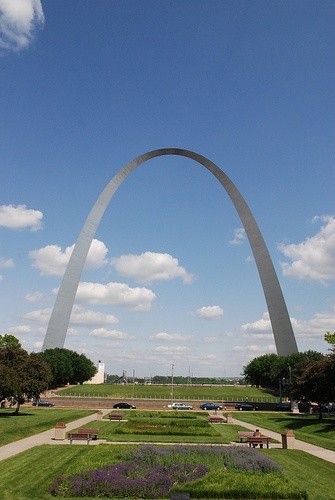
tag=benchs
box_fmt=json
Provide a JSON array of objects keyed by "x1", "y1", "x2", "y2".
[
  {"x1": 238, "y1": 431, "x2": 254, "y2": 443},
  {"x1": 79, "y1": 428, "x2": 100, "y2": 440},
  {"x1": 208, "y1": 415, "x2": 227, "y2": 424},
  {"x1": 247, "y1": 435, "x2": 272, "y2": 449},
  {"x1": 68, "y1": 433, "x2": 92, "y2": 445},
  {"x1": 109, "y1": 414, "x2": 122, "y2": 421}
]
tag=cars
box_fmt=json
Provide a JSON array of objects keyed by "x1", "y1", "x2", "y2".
[
  {"x1": 235, "y1": 401, "x2": 259, "y2": 411},
  {"x1": 200, "y1": 402, "x2": 224, "y2": 411},
  {"x1": 272, "y1": 403, "x2": 291, "y2": 411},
  {"x1": 113, "y1": 402, "x2": 137, "y2": 409},
  {"x1": 32, "y1": 401, "x2": 56, "y2": 408},
  {"x1": 171, "y1": 402, "x2": 193, "y2": 410},
  {"x1": 299, "y1": 402, "x2": 335, "y2": 413}
]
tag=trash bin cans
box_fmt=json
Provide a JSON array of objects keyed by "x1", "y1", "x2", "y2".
[
  {"x1": 55, "y1": 422, "x2": 66, "y2": 439},
  {"x1": 97, "y1": 410, "x2": 103, "y2": 420},
  {"x1": 227, "y1": 413, "x2": 232, "y2": 423},
  {"x1": 281, "y1": 430, "x2": 295, "y2": 449}
]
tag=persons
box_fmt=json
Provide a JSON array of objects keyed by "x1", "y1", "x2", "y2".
[{"x1": 252, "y1": 429, "x2": 263, "y2": 448}]
